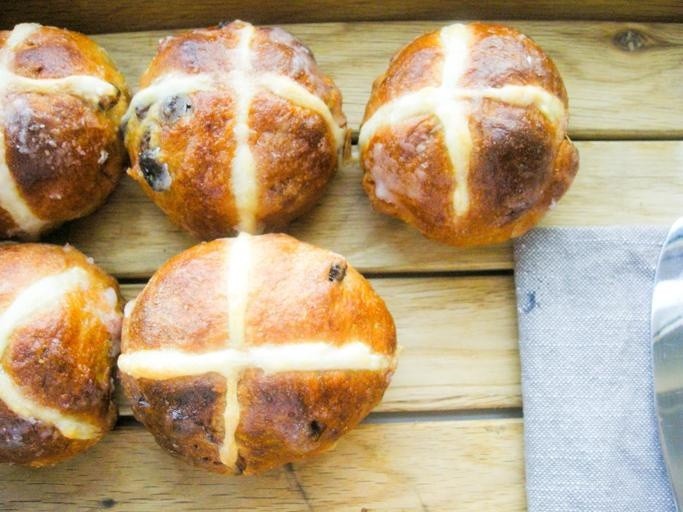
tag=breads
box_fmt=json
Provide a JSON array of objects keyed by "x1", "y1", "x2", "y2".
[
  {"x1": 358, "y1": 21, "x2": 581, "y2": 247},
  {"x1": 116, "y1": 233, "x2": 397, "y2": 478},
  {"x1": 0, "y1": 22, "x2": 132, "y2": 240},
  {"x1": 0, "y1": 241, "x2": 126, "y2": 469},
  {"x1": 122, "y1": 19, "x2": 353, "y2": 242}
]
